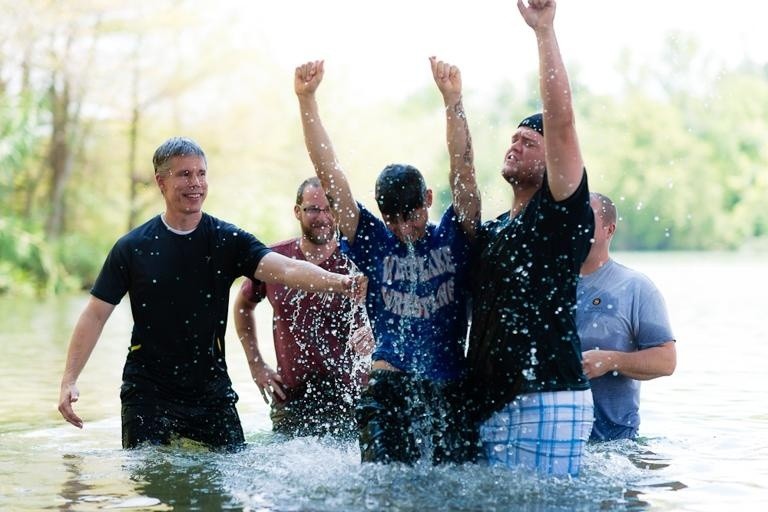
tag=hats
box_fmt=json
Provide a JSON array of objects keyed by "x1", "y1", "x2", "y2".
[{"x1": 518, "y1": 113, "x2": 543, "y2": 135}]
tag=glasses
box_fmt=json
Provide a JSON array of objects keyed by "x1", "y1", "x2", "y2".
[{"x1": 301, "y1": 204, "x2": 331, "y2": 214}]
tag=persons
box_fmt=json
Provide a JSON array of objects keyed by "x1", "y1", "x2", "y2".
[
  {"x1": 233, "y1": 174, "x2": 376, "y2": 441},
  {"x1": 293, "y1": 54, "x2": 482, "y2": 465},
  {"x1": 461, "y1": 0, "x2": 598, "y2": 482},
  {"x1": 570, "y1": 192, "x2": 681, "y2": 442},
  {"x1": 56, "y1": 135, "x2": 370, "y2": 453}
]
